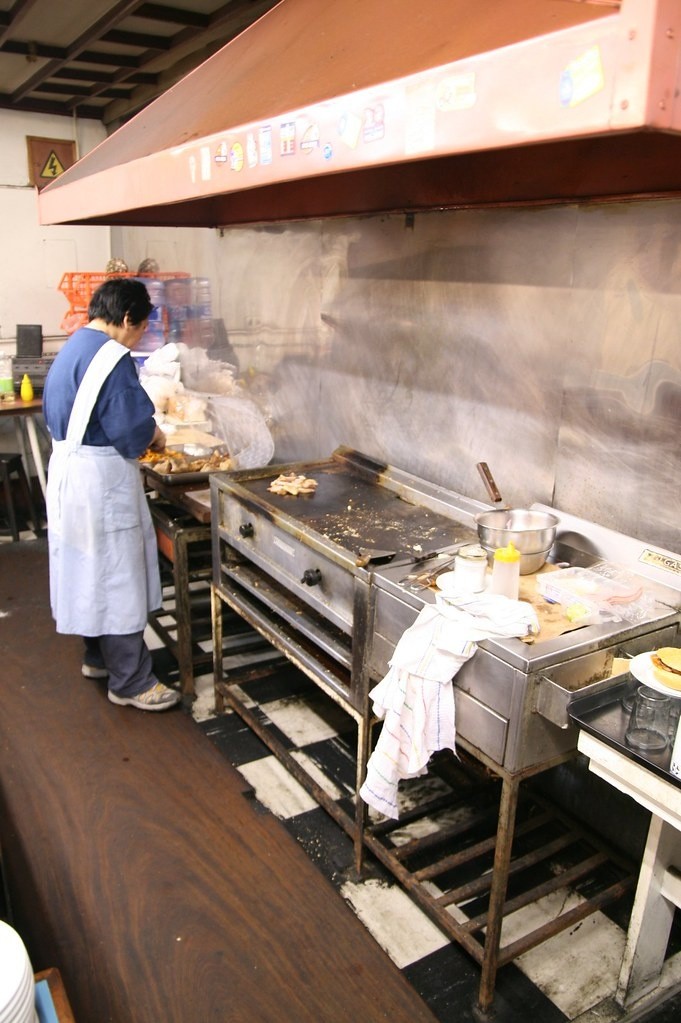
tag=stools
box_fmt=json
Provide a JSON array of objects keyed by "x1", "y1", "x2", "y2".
[{"x1": 0, "y1": 453, "x2": 40, "y2": 542}]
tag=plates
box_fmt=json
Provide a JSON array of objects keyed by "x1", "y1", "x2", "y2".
[
  {"x1": 436, "y1": 572, "x2": 492, "y2": 603},
  {"x1": 627, "y1": 650, "x2": 681, "y2": 701}
]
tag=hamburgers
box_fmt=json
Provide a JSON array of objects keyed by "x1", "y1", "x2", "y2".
[{"x1": 650, "y1": 647, "x2": 681, "y2": 693}]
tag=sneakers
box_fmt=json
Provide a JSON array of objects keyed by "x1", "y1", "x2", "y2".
[
  {"x1": 107, "y1": 682, "x2": 181, "y2": 711},
  {"x1": 81, "y1": 664, "x2": 109, "y2": 678}
]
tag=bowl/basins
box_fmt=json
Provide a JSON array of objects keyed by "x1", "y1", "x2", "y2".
[
  {"x1": 474, "y1": 508, "x2": 561, "y2": 554},
  {"x1": 480, "y1": 543, "x2": 556, "y2": 574}
]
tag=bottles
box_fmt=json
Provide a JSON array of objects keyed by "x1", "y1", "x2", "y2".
[
  {"x1": 454, "y1": 544, "x2": 487, "y2": 593},
  {"x1": 490, "y1": 541, "x2": 520, "y2": 603},
  {"x1": 162, "y1": 277, "x2": 211, "y2": 346},
  {"x1": 21, "y1": 373, "x2": 34, "y2": 402},
  {"x1": 119, "y1": 277, "x2": 164, "y2": 375}
]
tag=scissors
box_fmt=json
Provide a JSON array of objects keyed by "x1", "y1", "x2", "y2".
[{"x1": 397, "y1": 556, "x2": 455, "y2": 592}]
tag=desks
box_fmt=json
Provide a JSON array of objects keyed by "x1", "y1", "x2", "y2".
[
  {"x1": 576, "y1": 730, "x2": 681, "y2": 1014},
  {"x1": 0, "y1": 390, "x2": 47, "y2": 510}
]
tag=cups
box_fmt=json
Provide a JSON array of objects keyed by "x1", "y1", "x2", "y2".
[{"x1": 623, "y1": 675, "x2": 681, "y2": 750}]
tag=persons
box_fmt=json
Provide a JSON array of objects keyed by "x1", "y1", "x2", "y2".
[{"x1": 43, "y1": 278, "x2": 181, "y2": 710}]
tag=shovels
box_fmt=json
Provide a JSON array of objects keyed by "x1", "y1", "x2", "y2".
[
  {"x1": 410, "y1": 542, "x2": 471, "y2": 564},
  {"x1": 355, "y1": 547, "x2": 396, "y2": 567}
]
